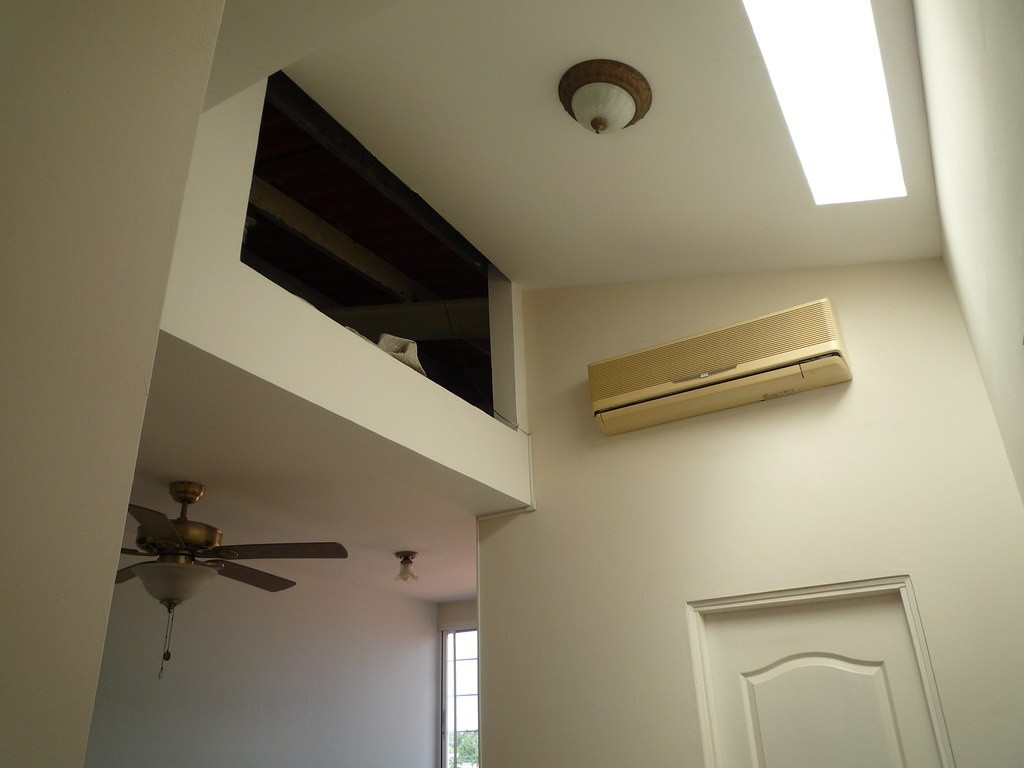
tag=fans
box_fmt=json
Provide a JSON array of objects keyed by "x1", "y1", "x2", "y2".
[{"x1": 115, "y1": 479, "x2": 350, "y2": 594}]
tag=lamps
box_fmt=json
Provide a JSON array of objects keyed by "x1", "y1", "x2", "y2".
[
  {"x1": 558, "y1": 58, "x2": 653, "y2": 136},
  {"x1": 392, "y1": 551, "x2": 420, "y2": 583},
  {"x1": 131, "y1": 561, "x2": 217, "y2": 682}
]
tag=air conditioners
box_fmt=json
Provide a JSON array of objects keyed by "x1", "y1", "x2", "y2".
[{"x1": 586, "y1": 298, "x2": 853, "y2": 436}]
